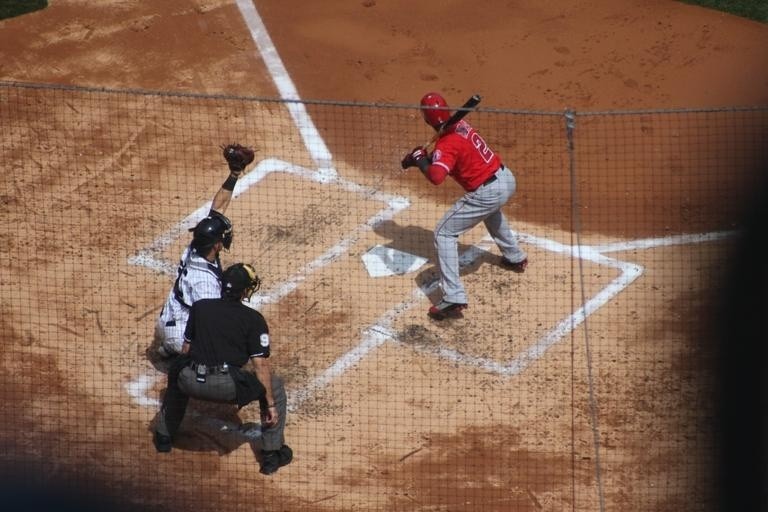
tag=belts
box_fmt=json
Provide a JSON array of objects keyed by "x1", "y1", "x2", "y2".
[
  {"x1": 188, "y1": 360, "x2": 222, "y2": 376},
  {"x1": 483, "y1": 164, "x2": 504, "y2": 185}
]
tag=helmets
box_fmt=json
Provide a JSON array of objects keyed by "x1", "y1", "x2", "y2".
[
  {"x1": 420, "y1": 93, "x2": 451, "y2": 127},
  {"x1": 221, "y1": 263, "x2": 261, "y2": 303},
  {"x1": 194, "y1": 209, "x2": 234, "y2": 250}
]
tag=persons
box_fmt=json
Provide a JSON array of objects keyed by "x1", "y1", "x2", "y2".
[
  {"x1": 401, "y1": 92, "x2": 529, "y2": 318},
  {"x1": 157, "y1": 143, "x2": 255, "y2": 357},
  {"x1": 152, "y1": 263, "x2": 293, "y2": 476}
]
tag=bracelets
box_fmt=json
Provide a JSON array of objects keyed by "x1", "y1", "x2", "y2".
[{"x1": 418, "y1": 158, "x2": 430, "y2": 172}]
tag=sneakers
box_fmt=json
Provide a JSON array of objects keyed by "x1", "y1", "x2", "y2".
[
  {"x1": 501, "y1": 255, "x2": 527, "y2": 270},
  {"x1": 154, "y1": 432, "x2": 171, "y2": 452},
  {"x1": 429, "y1": 300, "x2": 468, "y2": 318},
  {"x1": 262, "y1": 445, "x2": 292, "y2": 473}
]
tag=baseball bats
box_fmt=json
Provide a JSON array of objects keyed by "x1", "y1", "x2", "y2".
[{"x1": 422, "y1": 94, "x2": 481, "y2": 150}]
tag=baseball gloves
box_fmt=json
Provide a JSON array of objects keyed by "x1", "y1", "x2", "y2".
[{"x1": 219, "y1": 142, "x2": 260, "y2": 178}]
